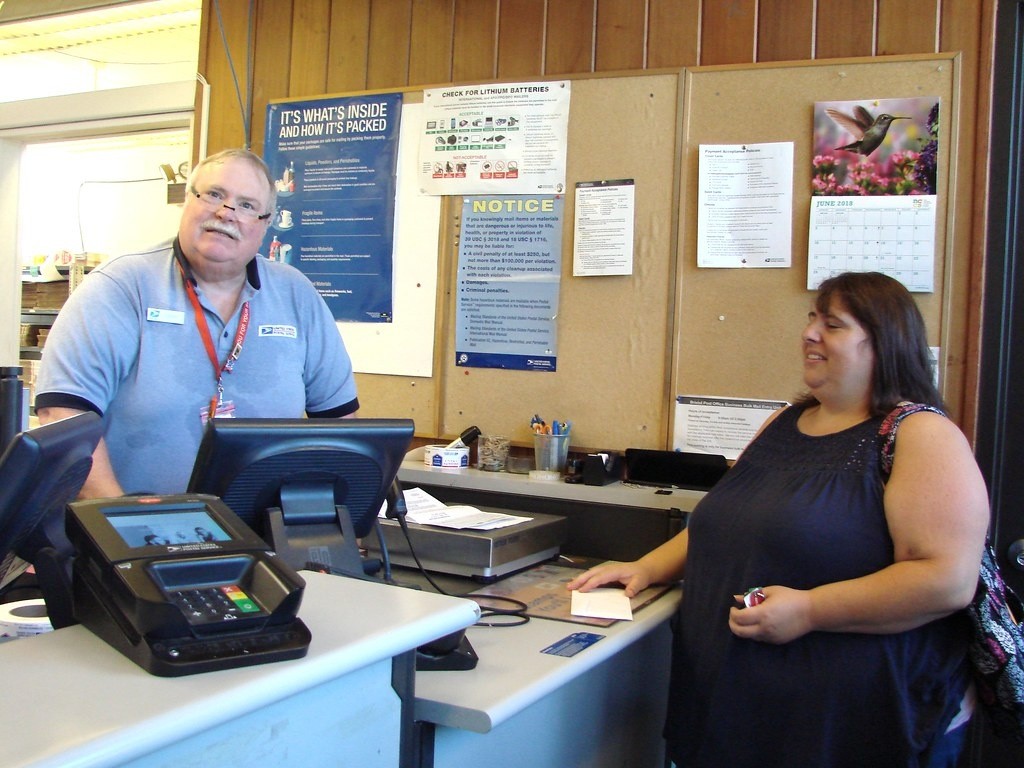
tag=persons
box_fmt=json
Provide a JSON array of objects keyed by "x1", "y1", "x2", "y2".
[
  {"x1": 565, "y1": 269, "x2": 1024, "y2": 768},
  {"x1": 34, "y1": 149, "x2": 361, "y2": 508}
]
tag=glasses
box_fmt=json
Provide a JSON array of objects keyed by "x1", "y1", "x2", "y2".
[{"x1": 190, "y1": 181, "x2": 273, "y2": 222}]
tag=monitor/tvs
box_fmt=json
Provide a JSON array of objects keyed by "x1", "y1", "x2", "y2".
[
  {"x1": 0, "y1": 410, "x2": 105, "y2": 633},
  {"x1": 186, "y1": 418, "x2": 415, "y2": 582}
]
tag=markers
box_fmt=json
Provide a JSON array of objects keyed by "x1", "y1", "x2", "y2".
[{"x1": 445, "y1": 426, "x2": 481, "y2": 448}]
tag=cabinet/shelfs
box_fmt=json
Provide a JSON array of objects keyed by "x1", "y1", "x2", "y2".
[{"x1": 18, "y1": 266, "x2": 95, "y2": 360}]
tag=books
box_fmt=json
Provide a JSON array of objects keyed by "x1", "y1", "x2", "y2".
[
  {"x1": 16, "y1": 356, "x2": 41, "y2": 406},
  {"x1": 27, "y1": 414, "x2": 41, "y2": 431},
  {"x1": 21, "y1": 273, "x2": 70, "y2": 311},
  {"x1": 18, "y1": 323, "x2": 52, "y2": 349}
]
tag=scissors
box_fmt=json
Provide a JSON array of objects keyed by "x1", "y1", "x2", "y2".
[{"x1": 534, "y1": 423, "x2": 552, "y2": 435}]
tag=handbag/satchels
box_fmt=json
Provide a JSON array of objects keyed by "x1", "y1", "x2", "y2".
[{"x1": 877, "y1": 401, "x2": 1024, "y2": 744}]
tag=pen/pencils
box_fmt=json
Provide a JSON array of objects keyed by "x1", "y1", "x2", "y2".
[
  {"x1": 530, "y1": 413, "x2": 573, "y2": 435},
  {"x1": 210, "y1": 394, "x2": 218, "y2": 418}
]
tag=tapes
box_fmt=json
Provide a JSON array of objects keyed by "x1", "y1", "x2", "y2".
[
  {"x1": 0, "y1": 599, "x2": 55, "y2": 638},
  {"x1": 423, "y1": 444, "x2": 471, "y2": 468}
]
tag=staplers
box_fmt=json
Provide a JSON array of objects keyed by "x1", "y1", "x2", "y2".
[{"x1": 564, "y1": 459, "x2": 585, "y2": 484}]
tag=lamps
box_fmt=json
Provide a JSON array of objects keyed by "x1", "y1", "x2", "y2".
[{"x1": 159, "y1": 163, "x2": 177, "y2": 183}]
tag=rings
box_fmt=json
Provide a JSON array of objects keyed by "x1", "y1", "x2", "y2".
[{"x1": 744, "y1": 585, "x2": 764, "y2": 595}]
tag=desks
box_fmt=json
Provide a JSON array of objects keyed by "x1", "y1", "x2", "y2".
[
  {"x1": 396, "y1": 461, "x2": 710, "y2": 562},
  {"x1": 377, "y1": 565, "x2": 682, "y2": 768},
  {"x1": 0, "y1": 571, "x2": 480, "y2": 768}
]
